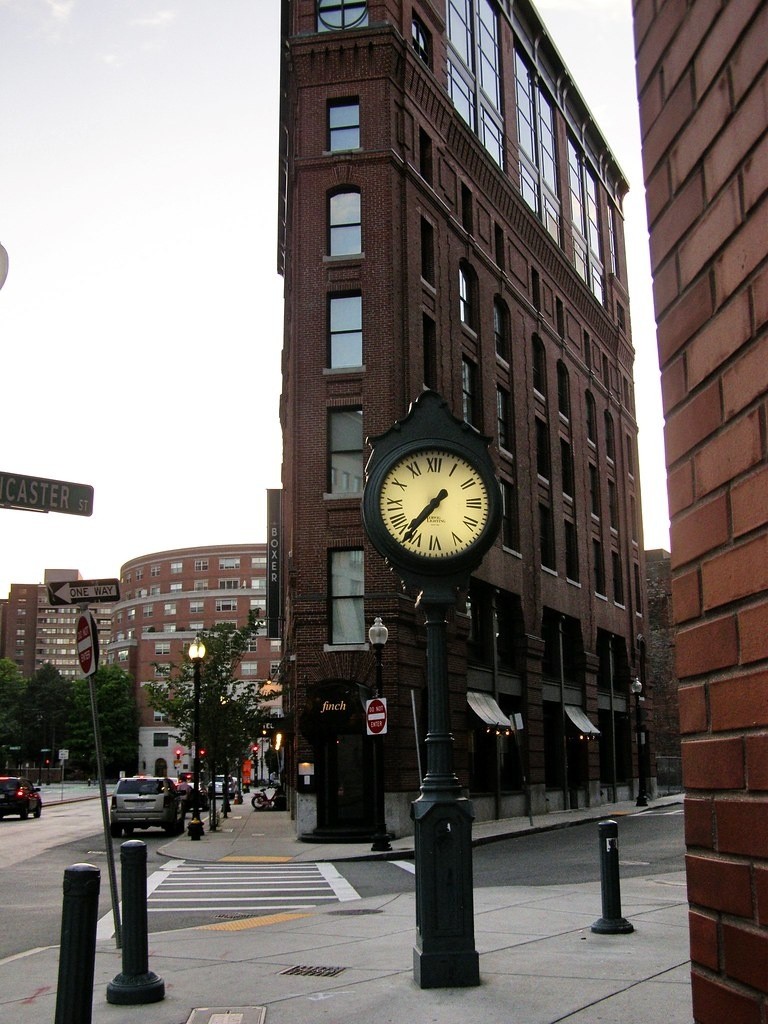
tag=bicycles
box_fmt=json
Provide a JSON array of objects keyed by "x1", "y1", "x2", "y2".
[{"x1": 251, "y1": 785, "x2": 285, "y2": 809}]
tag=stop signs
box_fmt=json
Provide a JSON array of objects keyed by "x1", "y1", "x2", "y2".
[
  {"x1": 365, "y1": 698, "x2": 389, "y2": 736},
  {"x1": 75, "y1": 612, "x2": 97, "y2": 680}
]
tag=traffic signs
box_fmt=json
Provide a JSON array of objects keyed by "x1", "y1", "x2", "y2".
[
  {"x1": 0, "y1": 471, "x2": 94, "y2": 517},
  {"x1": 47, "y1": 578, "x2": 122, "y2": 608}
]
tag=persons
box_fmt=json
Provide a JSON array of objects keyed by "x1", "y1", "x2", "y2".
[{"x1": 176, "y1": 776, "x2": 191, "y2": 818}]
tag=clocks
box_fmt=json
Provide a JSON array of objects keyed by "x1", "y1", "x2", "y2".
[{"x1": 360, "y1": 437, "x2": 503, "y2": 573}]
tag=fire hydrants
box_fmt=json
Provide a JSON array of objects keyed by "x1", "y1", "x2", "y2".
[
  {"x1": 189, "y1": 818, "x2": 201, "y2": 840},
  {"x1": 88, "y1": 778, "x2": 91, "y2": 786}
]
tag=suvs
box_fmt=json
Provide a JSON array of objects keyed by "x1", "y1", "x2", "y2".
[
  {"x1": 208, "y1": 775, "x2": 235, "y2": 798},
  {"x1": 0, "y1": 776, "x2": 42, "y2": 820},
  {"x1": 110, "y1": 777, "x2": 185, "y2": 837}
]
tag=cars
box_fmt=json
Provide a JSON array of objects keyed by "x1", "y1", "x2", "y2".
[{"x1": 185, "y1": 782, "x2": 208, "y2": 810}]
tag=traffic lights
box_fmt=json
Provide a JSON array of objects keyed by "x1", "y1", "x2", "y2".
[
  {"x1": 177, "y1": 750, "x2": 180, "y2": 759},
  {"x1": 253, "y1": 746, "x2": 259, "y2": 758},
  {"x1": 46, "y1": 759, "x2": 50, "y2": 767},
  {"x1": 200, "y1": 750, "x2": 205, "y2": 760}
]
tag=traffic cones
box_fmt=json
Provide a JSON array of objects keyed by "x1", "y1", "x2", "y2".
[{"x1": 233, "y1": 791, "x2": 239, "y2": 805}]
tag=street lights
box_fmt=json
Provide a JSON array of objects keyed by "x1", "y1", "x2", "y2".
[
  {"x1": 629, "y1": 677, "x2": 649, "y2": 807},
  {"x1": 368, "y1": 612, "x2": 393, "y2": 852},
  {"x1": 187, "y1": 632, "x2": 205, "y2": 833}
]
